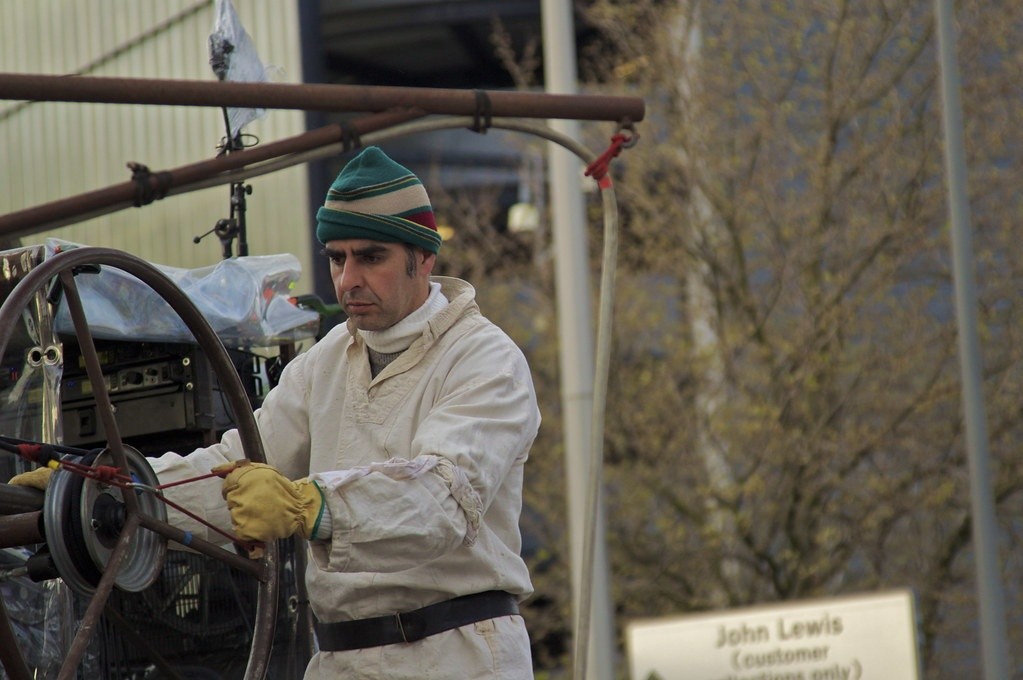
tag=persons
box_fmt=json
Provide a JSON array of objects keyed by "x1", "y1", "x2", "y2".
[{"x1": 11, "y1": 146, "x2": 540, "y2": 679}]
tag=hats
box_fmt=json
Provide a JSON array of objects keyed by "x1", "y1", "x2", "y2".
[{"x1": 314, "y1": 146, "x2": 443, "y2": 258}]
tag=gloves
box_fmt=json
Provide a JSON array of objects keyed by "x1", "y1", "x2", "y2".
[
  {"x1": 5, "y1": 465, "x2": 54, "y2": 491},
  {"x1": 210, "y1": 459, "x2": 325, "y2": 551}
]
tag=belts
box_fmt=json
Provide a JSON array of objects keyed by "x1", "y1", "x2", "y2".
[{"x1": 311, "y1": 589, "x2": 520, "y2": 653}]
